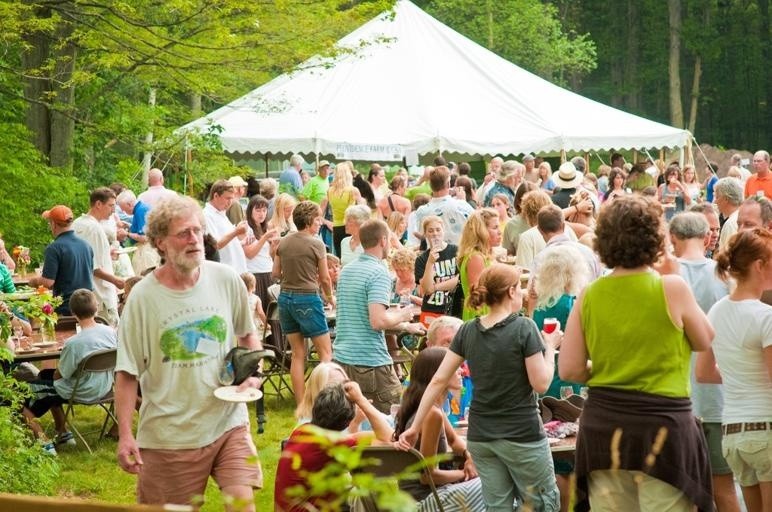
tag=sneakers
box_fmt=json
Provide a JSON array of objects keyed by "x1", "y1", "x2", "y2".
[
  {"x1": 103, "y1": 432, "x2": 119, "y2": 441},
  {"x1": 45, "y1": 444, "x2": 57, "y2": 456},
  {"x1": 55, "y1": 431, "x2": 76, "y2": 447}
]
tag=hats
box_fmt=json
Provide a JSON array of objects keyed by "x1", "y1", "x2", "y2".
[
  {"x1": 319, "y1": 160, "x2": 329, "y2": 168},
  {"x1": 217, "y1": 348, "x2": 276, "y2": 386},
  {"x1": 552, "y1": 161, "x2": 583, "y2": 189},
  {"x1": 228, "y1": 176, "x2": 248, "y2": 187},
  {"x1": 43, "y1": 205, "x2": 73, "y2": 224},
  {"x1": 538, "y1": 394, "x2": 585, "y2": 423}
]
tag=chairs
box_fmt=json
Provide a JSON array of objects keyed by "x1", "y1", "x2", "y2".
[
  {"x1": 6, "y1": 267, "x2": 118, "y2": 455},
  {"x1": 259, "y1": 301, "x2": 296, "y2": 408},
  {"x1": 350, "y1": 448, "x2": 446, "y2": 512}
]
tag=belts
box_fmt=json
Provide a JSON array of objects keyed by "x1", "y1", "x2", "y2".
[{"x1": 722, "y1": 422, "x2": 772, "y2": 434}]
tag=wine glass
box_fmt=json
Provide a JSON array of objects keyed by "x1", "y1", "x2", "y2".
[
  {"x1": 464, "y1": 406, "x2": 469, "y2": 422},
  {"x1": 580, "y1": 386, "x2": 590, "y2": 400},
  {"x1": 559, "y1": 385, "x2": 574, "y2": 400},
  {"x1": 216, "y1": 361, "x2": 235, "y2": 386},
  {"x1": 543, "y1": 318, "x2": 560, "y2": 354},
  {"x1": 13, "y1": 326, "x2": 23, "y2": 344}
]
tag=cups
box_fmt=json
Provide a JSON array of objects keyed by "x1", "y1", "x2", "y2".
[
  {"x1": 431, "y1": 238, "x2": 442, "y2": 251},
  {"x1": 20, "y1": 337, "x2": 33, "y2": 350},
  {"x1": 39, "y1": 262, "x2": 45, "y2": 268},
  {"x1": 34, "y1": 267, "x2": 42, "y2": 276},
  {"x1": 399, "y1": 296, "x2": 410, "y2": 309},
  {"x1": 9, "y1": 269, "x2": 15, "y2": 276}
]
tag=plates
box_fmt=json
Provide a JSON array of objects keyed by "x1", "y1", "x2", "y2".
[
  {"x1": 213, "y1": 385, "x2": 263, "y2": 402},
  {"x1": 33, "y1": 341, "x2": 57, "y2": 347},
  {"x1": 453, "y1": 420, "x2": 469, "y2": 427},
  {"x1": 115, "y1": 247, "x2": 137, "y2": 254},
  {"x1": 16, "y1": 347, "x2": 39, "y2": 352}
]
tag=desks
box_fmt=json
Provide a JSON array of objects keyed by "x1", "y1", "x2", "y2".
[{"x1": 307, "y1": 309, "x2": 336, "y2": 364}]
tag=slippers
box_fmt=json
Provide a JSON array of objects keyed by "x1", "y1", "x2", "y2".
[{"x1": 398, "y1": 375, "x2": 406, "y2": 382}]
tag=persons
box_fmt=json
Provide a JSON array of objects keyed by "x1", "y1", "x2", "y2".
[{"x1": 1, "y1": 150, "x2": 772, "y2": 512}]
